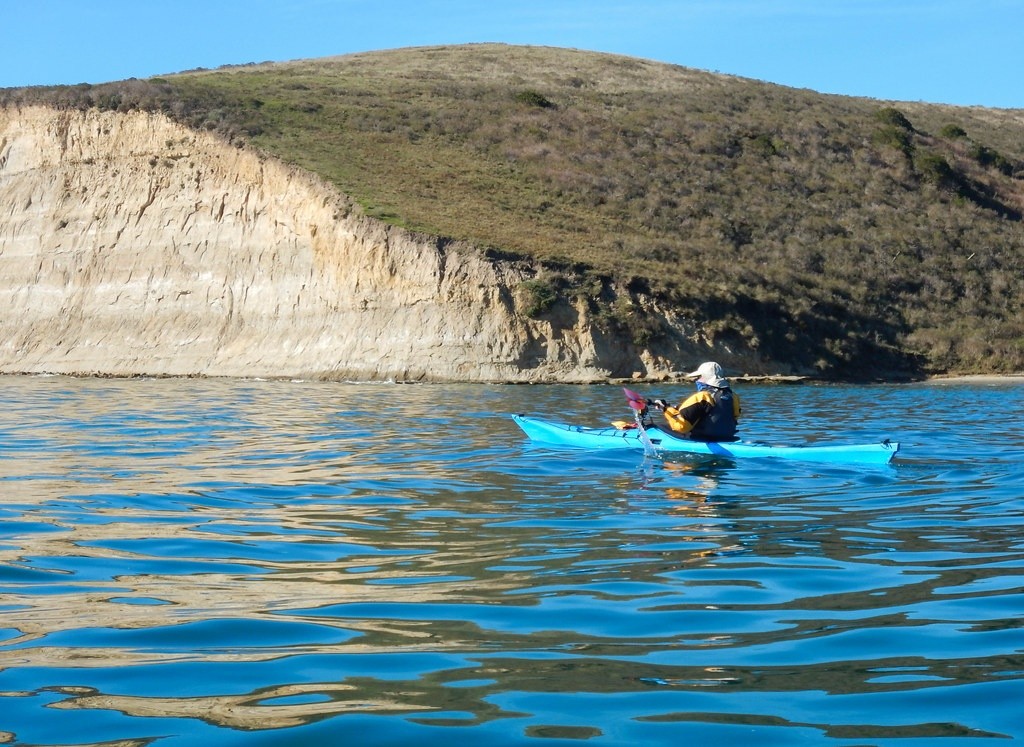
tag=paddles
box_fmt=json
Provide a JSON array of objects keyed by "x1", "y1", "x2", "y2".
[{"x1": 623, "y1": 386, "x2": 660, "y2": 410}]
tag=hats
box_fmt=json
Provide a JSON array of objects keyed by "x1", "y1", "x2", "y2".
[{"x1": 686, "y1": 362, "x2": 730, "y2": 388}]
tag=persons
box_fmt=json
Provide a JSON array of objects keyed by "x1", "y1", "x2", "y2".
[{"x1": 655, "y1": 362, "x2": 741, "y2": 441}]
